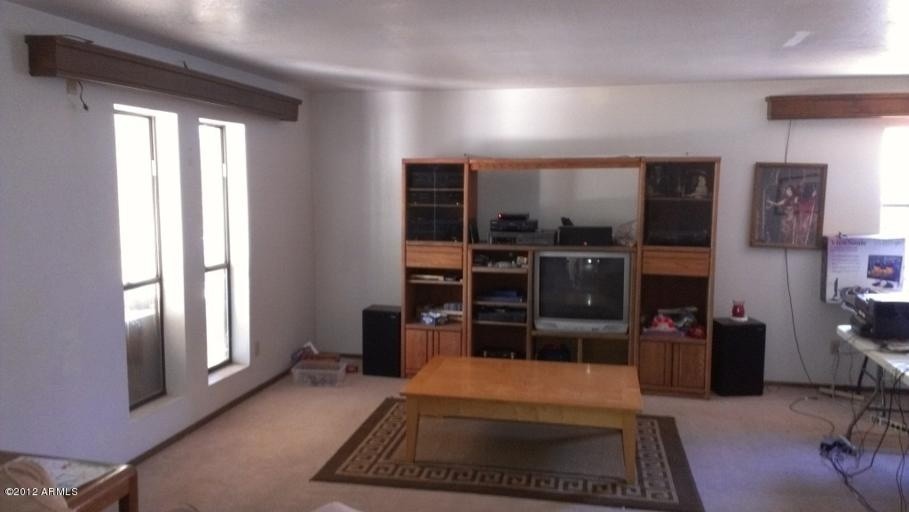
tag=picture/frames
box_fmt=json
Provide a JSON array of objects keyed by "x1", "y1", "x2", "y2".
[{"x1": 747, "y1": 161, "x2": 828, "y2": 250}]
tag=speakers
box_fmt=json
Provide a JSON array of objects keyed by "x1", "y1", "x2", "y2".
[
  {"x1": 361, "y1": 303, "x2": 402, "y2": 376},
  {"x1": 711, "y1": 315, "x2": 766, "y2": 397}
]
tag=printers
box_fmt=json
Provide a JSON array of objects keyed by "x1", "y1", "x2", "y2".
[{"x1": 850, "y1": 291, "x2": 909, "y2": 339}]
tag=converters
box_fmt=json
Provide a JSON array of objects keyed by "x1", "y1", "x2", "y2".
[{"x1": 820, "y1": 436, "x2": 835, "y2": 449}]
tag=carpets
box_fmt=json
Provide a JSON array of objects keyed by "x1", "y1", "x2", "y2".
[{"x1": 311, "y1": 396, "x2": 706, "y2": 512}]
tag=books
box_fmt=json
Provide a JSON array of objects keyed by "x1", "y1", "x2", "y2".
[{"x1": 473, "y1": 283, "x2": 526, "y2": 305}]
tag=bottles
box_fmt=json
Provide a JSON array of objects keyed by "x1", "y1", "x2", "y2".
[{"x1": 732, "y1": 301, "x2": 745, "y2": 317}]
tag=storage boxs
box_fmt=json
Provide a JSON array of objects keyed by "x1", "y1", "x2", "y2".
[{"x1": 290, "y1": 359, "x2": 348, "y2": 389}]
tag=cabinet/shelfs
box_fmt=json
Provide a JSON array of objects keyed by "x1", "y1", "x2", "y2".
[{"x1": 396, "y1": 155, "x2": 724, "y2": 402}]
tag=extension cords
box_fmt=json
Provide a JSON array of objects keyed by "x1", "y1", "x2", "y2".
[{"x1": 836, "y1": 436, "x2": 857, "y2": 457}]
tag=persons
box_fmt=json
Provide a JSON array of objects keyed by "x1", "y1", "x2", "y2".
[{"x1": 765, "y1": 182, "x2": 799, "y2": 245}]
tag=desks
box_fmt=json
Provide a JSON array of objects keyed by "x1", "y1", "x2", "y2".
[
  {"x1": 398, "y1": 355, "x2": 644, "y2": 486},
  {"x1": 1, "y1": 450, "x2": 139, "y2": 512},
  {"x1": 834, "y1": 323, "x2": 909, "y2": 456}
]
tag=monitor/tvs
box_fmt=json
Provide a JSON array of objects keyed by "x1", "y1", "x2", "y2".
[{"x1": 532, "y1": 249, "x2": 630, "y2": 334}]
